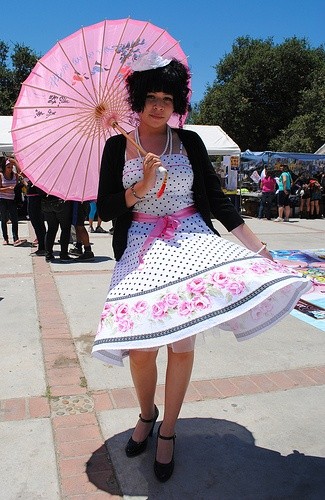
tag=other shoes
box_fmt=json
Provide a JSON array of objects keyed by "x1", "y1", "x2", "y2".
[
  {"x1": 3, "y1": 241, "x2": 9, "y2": 245},
  {"x1": 306, "y1": 212, "x2": 310, "y2": 217},
  {"x1": 71, "y1": 248, "x2": 82, "y2": 256},
  {"x1": 73, "y1": 251, "x2": 95, "y2": 261},
  {"x1": 46, "y1": 255, "x2": 55, "y2": 262},
  {"x1": 58, "y1": 239, "x2": 73, "y2": 244},
  {"x1": 96, "y1": 226, "x2": 106, "y2": 233},
  {"x1": 299, "y1": 213, "x2": 303, "y2": 219},
  {"x1": 32, "y1": 239, "x2": 39, "y2": 247},
  {"x1": 31, "y1": 248, "x2": 46, "y2": 256},
  {"x1": 273, "y1": 217, "x2": 285, "y2": 222},
  {"x1": 88, "y1": 227, "x2": 94, "y2": 233},
  {"x1": 14, "y1": 239, "x2": 27, "y2": 247},
  {"x1": 282, "y1": 217, "x2": 289, "y2": 222},
  {"x1": 60, "y1": 251, "x2": 74, "y2": 261}
]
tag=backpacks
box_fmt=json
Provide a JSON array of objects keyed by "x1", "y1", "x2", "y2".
[{"x1": 312, "y1": 183, "x2": 320, "y2": 191}]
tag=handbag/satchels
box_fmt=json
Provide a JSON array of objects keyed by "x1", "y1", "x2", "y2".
[{"x1": 250, "y1": 170, "x2": 261, "y2": 183}]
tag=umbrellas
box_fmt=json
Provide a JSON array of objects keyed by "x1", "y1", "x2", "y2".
[{"x1": 8, "y1": 15, "x2": 195, "y2": 204}]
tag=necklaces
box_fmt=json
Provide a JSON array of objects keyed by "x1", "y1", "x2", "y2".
[{"x1": 135, "y1": 125, "x2": 173, "y2": 158}]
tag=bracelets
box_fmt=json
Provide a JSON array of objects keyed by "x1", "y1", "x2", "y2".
[
  {"x1": 257, "y1": 242, "x2": 267, "y2": 254},
  {"x1": 131, "y1": 183, "x2": 141, "y2": 199}
]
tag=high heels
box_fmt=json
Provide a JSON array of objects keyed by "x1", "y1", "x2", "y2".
[
  {"x1": 125, "y1": 404, "x2": 159, "y2": 458},
  {"x1": 154, "y1": 421, "x2": 177, "y2": 483}
]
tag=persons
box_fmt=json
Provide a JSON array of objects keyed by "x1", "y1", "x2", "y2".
[
  {"x1": 258, "y1": 163, "x2": 325, "y2": 222},
  {"x1": 91, "y1": 50, "x2": 312, "y2": 483},
  {"x1": 0, "y1": 157, "x2": 114, "y2": 264}
]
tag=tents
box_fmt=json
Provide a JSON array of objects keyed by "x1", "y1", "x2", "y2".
[
  {"x1": 0, "y1": 115, "x2": 14, "y2": 154},
  {"x1": 239, "y1": 149, "x2": 325, "y2": 215},
  {"x1": 182, "y1": 125, "x2": 241, "y2": 215}
]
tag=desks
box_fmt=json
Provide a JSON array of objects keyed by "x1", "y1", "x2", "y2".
[{"x1": 242, "y1": 193, "x2": 296, "y2": 217}]
